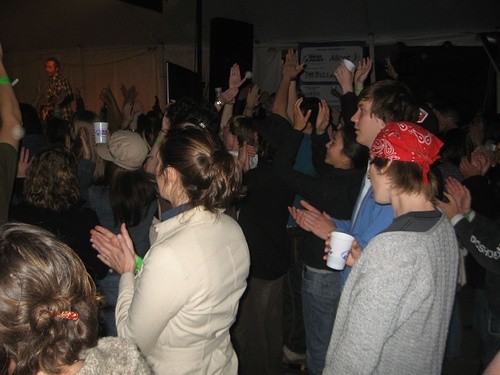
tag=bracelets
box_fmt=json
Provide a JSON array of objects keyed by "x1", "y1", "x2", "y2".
[
  {"x1": 162, "y1": 128, "x2": 168, "y2": 133},
  {"x1": 134, "y1": 256, "x2": 142, "y2": 274},
  {"x1": 355, "y1": 88, "x2": 363, "y2": 93},
  {"x1": 465, "y1": 211, "x2": 472, "y2": 220},
  {"x1": 156, "y1": 138, "x2": 164, "y2": 145},
  {"x1": 130, "y1": 128, "x2": 137, "y2": 132}
]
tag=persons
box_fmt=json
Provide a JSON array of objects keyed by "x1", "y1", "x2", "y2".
[
  {"x1": 0, "y1": 47, "x2": 500, "y2": 375},
  {"x1": 321, "y1": 122, "x2": 460, "y2": 375}
]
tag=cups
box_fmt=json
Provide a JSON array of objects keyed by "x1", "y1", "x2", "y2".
[
  {"x1": 344, "y1": 59, "x2": 355, "y2": 71},
  {"x1": 327, "y1": 231, "x2": 355, "y2": 270},
  {"x1": 93, "y1": 122, "x2": 108, "y2": 143}
]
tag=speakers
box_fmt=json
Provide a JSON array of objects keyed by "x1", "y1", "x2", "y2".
[
  {"x1": 166, "y1": 61, "x2": 203, "y2": 106},
  {"x1": 210, "y1": 18, "x2": 254, "y2": 88}
]
